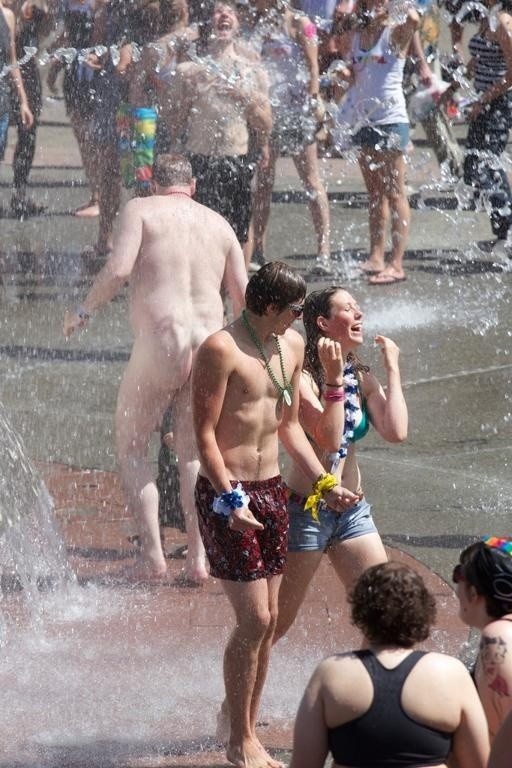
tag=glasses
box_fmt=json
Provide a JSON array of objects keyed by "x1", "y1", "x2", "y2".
[
  {"x1": 453, "y1": 565, "x2": 467, "y2": 584},
  {"x1": 284, "y1": 304, "x2": 305, "y2": 318}
]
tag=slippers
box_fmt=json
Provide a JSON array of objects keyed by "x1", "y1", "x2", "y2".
[{"x1": 312, "y1": 256, "x2": 406, "y2": 284}]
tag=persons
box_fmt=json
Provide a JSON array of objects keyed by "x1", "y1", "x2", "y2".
[{"x1": 1, "y1": 0, "x2": 512, "y2": 767}]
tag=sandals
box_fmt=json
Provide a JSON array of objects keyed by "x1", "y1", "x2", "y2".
[
  {"x1": 126, "y1": 531, "x2": 165, "y2": 552},
  {"x1": 167, "y1": 544, "x2": 188, "y2": 558}
]
